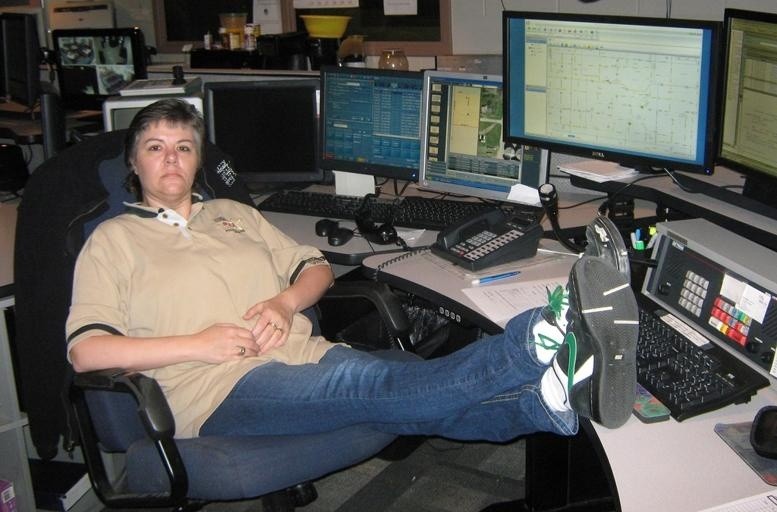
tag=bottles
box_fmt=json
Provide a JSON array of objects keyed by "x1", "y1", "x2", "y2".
[
  {"x1": 203, "y1": 14, "x2": 259, "y2": 51},
  {"x1": 379, "y1": 49, "x2": 409, "y2": 70}
]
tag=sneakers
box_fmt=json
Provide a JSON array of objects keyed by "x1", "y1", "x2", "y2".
[{"x1": 540, "y1": 218, "x2": 641, "y2": 428}]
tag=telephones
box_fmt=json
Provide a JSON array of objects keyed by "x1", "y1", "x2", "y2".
[
  {"x1": 353, "y1": 209, "x2": 398, "y2": 245},
  {"x1": 430, "y1": 207, "x2": 544, "y2": 272}
]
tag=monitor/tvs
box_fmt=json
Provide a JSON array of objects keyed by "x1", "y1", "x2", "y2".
[
  {"x1": 52, "y1": 27, "x2": 147, "y2": 109},
  {"x1": 203, "y1": 80, "x2": 324, "y2": 182},
  {"x1": 419, "y1": 68, "x2": 551, "y2": 207},
  {"x1": 0, "y1": 13, "x2": 40, "y2": 109},
  {"x1": 717, "y1": 8, "x2": 777, "y2": 184},
  {"x1": 100, "y1": 97, "x2": 205, "y2": 133},
  {"x1": 321, "y1": 65, "x2": 424, "y2": 181},
  {"x1": 501, "y1": 10, "x2": 728, "y2": 176}
]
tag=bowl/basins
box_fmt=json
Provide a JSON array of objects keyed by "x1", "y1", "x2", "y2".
[{"x1": 301, "y1": 15, "x2": 350, "y2": 38}]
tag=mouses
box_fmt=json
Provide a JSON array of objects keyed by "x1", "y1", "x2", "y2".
[
  {"x1": 315, "y1": 219, "x2": 337, "y2": 236},
  {"x1": 375, "y1": 223, "x2": 397, "y2": 244},
  {"x1": 750, "y1": 406, "x2": 777, "y2": 460},
  {"x1": 328, "y1": 227, "x2": 354, "y2": 246}
]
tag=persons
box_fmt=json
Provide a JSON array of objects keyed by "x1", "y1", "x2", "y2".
[{"x1": 64, "y1": 96, "x2": 639, "y2": 447}]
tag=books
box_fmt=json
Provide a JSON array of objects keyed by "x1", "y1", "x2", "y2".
[{"x1": 117, "y1": 75, "x2": 202, "y2": 96}]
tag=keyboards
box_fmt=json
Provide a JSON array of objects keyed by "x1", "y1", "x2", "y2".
[
  {"x1": 629, "y1": 307, "x2": 771, "y2": 422},
  {"x1": 393, "y1": 196, "x2": 544, "y2": 232},
  {"x1": 256, "y1": 190, "x2": 400, "y2": 223}
]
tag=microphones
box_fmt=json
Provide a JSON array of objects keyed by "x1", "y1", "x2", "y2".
[{"x1": 537, "y1": 183, "x2": 657, "y2": 269}]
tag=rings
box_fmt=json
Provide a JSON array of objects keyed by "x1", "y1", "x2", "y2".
[
  {"x1": 239, "y1": 346, "x2": 245, "y2": 357},
  {"x1": 268, "y1": 322, "x2": 276, "y2": 330},
  {"x1": 276, "y1": 325, "x2": 284, "y2": 333}
]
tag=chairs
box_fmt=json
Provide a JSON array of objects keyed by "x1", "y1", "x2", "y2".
[{"x1": 14, "y1": 124, "x2": 413, "y2": 512}]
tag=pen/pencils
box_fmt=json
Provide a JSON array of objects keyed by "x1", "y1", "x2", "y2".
[
  {"x1": 630, "y1": 224, "x2": 658, "y2": 252},
  {"x1": 472, "y1": 271, "x2": 521, "y2": 285}
]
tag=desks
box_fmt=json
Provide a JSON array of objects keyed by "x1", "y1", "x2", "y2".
[
  {"x1": 363, "y1": 191, "x2": 776, "y2": 511},
  {"x1": 0, "y1": 166, "x2": 507, "y2": 512}
]
